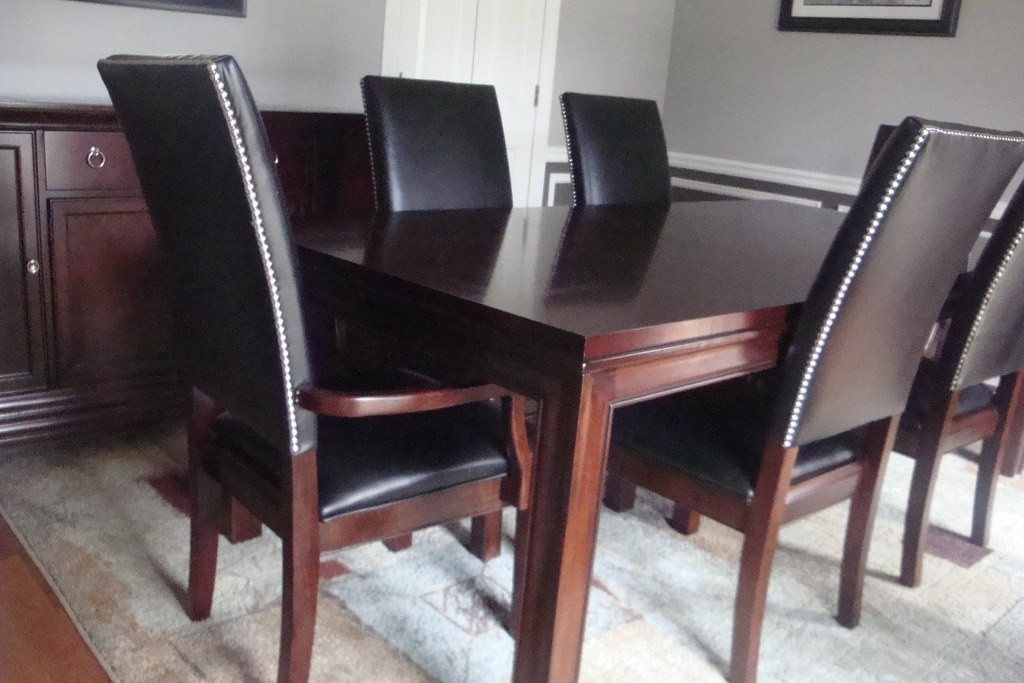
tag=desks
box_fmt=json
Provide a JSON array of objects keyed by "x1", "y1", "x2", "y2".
[{"x1": 287, "y1": 200, "x2": 1023, "y2": 683}]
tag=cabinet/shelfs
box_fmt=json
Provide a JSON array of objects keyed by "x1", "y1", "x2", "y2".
[{"x1": 0, "y1": 119, "x2": 375, "y2": 447}]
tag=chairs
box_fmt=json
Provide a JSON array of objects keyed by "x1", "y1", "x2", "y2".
[
  {"x1": 96, "y1": 54, "x2": 534, "y2": 683},
  {"x1": 560, "y1": 92, "x2": 700, "y2": 534},
  {"x1": 361, "y1": 74, "x2": 541, "y2": 563},
  {"x1": 891, "y1": 176, "x2": 1023, "y2": 588},
  {"x1": 602, "y1": 115, "x2": 1024, "y2": 683}
]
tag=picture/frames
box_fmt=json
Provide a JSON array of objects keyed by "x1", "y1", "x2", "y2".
[{"x1": 777, "y1": 0, "x2": 962, "y2": 38}]
